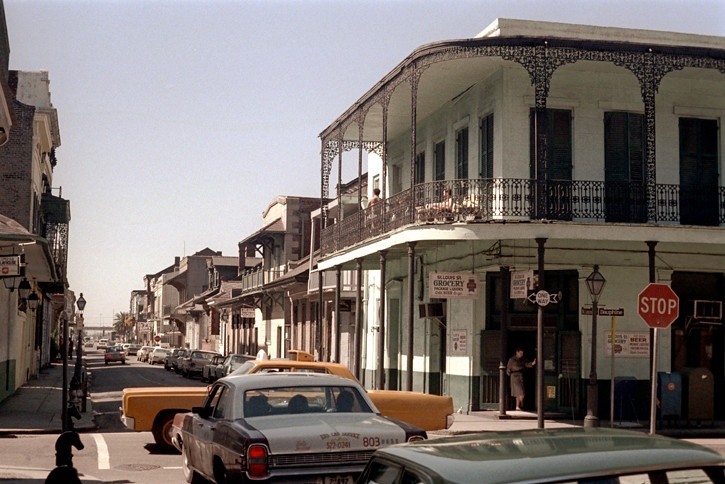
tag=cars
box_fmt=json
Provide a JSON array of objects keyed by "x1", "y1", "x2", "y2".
[
  {"x1": 172, "y1": 372, "x2": 429, "y2": 484},
  {"x1": 118, "y1": 350, "x2": 455, "y2": 455},
  {"x1": 83, "y1": 337, "x2": 256, "y2": 384},
  {"x1": 103, "y1": 346, "x2": 126, "y2": 366},
  {"x1": 352, "y1": 427, "x2": 725, "y2": 484}
]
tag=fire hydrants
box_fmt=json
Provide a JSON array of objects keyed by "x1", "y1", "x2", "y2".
[{"x1": 67, "y1": 376, "x2": 81, "y2": 413}]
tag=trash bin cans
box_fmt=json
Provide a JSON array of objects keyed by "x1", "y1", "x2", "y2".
[
  {"x1": 681, "y1": 366, "x2": 715, "y2": 426},
  {"x1": 656, "y1": 371, "x2": 682, "y2": 429},
  {"x1": 615, "y1": 376, "x2": 640, "y2": 421}
]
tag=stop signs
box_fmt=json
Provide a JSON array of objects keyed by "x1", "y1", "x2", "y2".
[
  {"x1": 155, "y1": 336, "x2": 160, "y2": 342},
  {"x1": 636, "y1": 283, "x2": 679, "y2": 329}
]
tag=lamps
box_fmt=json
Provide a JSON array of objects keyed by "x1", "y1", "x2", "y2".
[{"x1": 3, "y1": 277, "x2": 40, "y2": 312}]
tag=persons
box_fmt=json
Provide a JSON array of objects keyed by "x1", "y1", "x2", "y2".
[
  {"x1": 365, "y1": 187, "x2": 382, "y2": 228},
  {"x1": 433, "y1": 187, "x2": 453, "y2": 212},
  {"x1": 69, "y1": 337, "x2": 73, "y2": 360},
  {"x1": 59, "y1": 344, "x2": 63, "y2": 359},
  {"x1": 506, "y1": 348, "x2": 536, "y2": 411}
]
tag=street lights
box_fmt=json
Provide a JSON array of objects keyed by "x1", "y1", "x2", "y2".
[
  {"x1": 582, "y1": 263, "x2": 606, "y2": 427},
  {"x1": 74, "y1": 292, "x2": 87, "y2": 387}
]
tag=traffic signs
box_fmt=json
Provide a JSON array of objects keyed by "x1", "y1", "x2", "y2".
[{"x1": 527, "y1": 290, "x2": 558, "y2": 307}]
